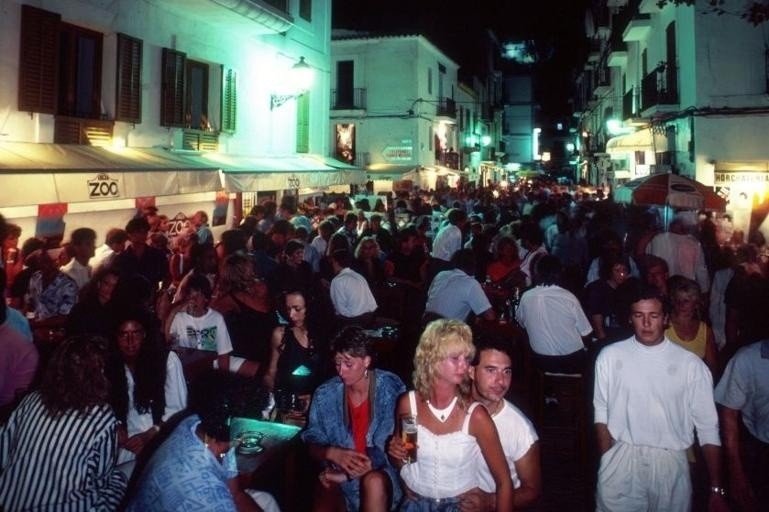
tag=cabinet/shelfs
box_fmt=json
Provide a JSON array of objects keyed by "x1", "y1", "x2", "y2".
[{"x1": 400, "y1": 415, "x2": 418, "y2": 464}]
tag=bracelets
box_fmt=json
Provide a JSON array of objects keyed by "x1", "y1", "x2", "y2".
[{"x1": 238, "y1": 431, "x2": 263, "y2": 447}]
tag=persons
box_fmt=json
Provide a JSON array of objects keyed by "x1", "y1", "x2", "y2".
[{"x1": 0, "y1": 178, "x2": 768, "y2": 512}]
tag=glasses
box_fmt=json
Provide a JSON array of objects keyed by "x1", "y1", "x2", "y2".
[{"x1": 270, "y1": 56, "x2": 313, "y2": 109}]
